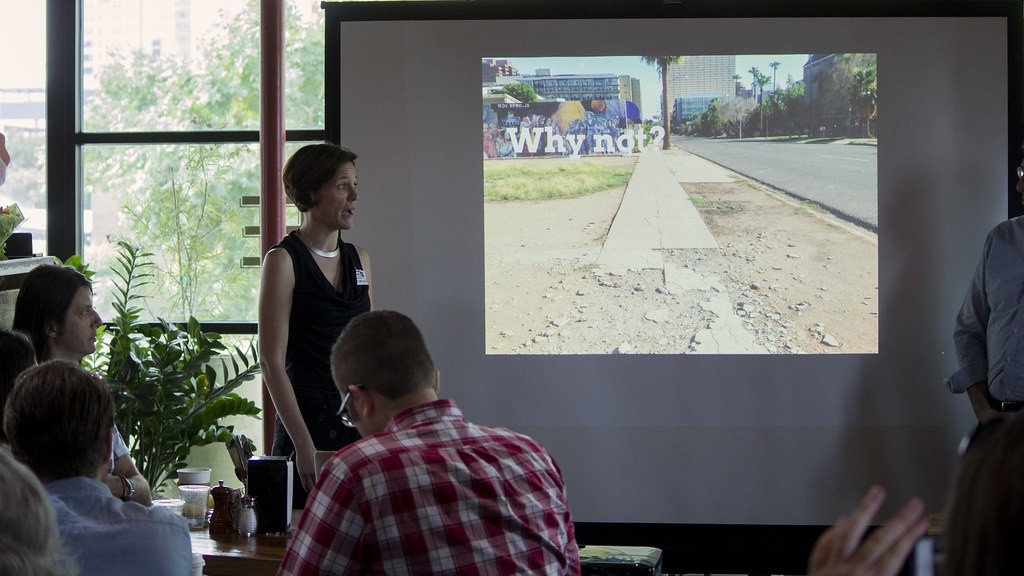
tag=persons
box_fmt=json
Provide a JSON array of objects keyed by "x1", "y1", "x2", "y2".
[
  {"x1": 13, "y1": 265, "x2": 153, "y2": 508},
  {"x1": 3, "y1": 360, "x2": 196, "y2": 575},
  {"x1": 276, "y1": 311, "x2": 581, "y2": 576},
  {"x1": 807, "y1": 415, "x2": 1023, "y2": 576},
  {"x1": 0, "y1": 441, "x2": 83, "y2": 576},
  {"x1": 260, "y1": 143, "x2": 375, "y2": 512},
  {"x1": 945, "y1": 146, "x2": 1024, "y2": 421},
  {"x1": 0, "y1": 327, "x2": 40, "y2": 444}
]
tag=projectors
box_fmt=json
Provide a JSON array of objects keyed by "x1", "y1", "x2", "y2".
[{"x1": 577, "y1": 545, "x2": 663, "y2": 576}]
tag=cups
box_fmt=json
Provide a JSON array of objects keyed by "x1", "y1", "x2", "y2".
[
  {"x1": 178, "y1": 484, "x2": 210, "y2": 529},
  {"x1": 152, "y1": 499, "x2": 185, "y2": 514},
  {"x1": 177, "y1": 468, "x2": 212, "y2": 486}
]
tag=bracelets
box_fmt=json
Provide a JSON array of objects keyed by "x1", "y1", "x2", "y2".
[{"x1": 119, "y1": 474, "x2": 135, "y2": 501}]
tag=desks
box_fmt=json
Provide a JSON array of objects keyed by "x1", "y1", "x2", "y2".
[{"x1": 189, "y1": 509, "x2": 309, "y2": 576}]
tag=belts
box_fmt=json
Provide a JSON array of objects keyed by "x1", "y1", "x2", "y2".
[{"x1": 986, "y1": 393, "x2": 1024, "y2": 413}]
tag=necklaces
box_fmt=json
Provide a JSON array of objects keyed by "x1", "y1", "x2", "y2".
[{"x1": 300, "y1": 236, "x2": 341, "y2": 258}]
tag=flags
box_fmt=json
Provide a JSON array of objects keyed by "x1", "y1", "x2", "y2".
[{"x1": 627, "y1": 99, "x2": 640, "y2": 123}]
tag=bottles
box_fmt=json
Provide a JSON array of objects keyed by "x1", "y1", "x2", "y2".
[{"x1": 238, "y1": 496, "x2": 258, "y2": 537}]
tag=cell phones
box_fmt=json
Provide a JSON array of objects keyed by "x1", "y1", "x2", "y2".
[{"x1": 910, "y1": 533, "x2": 948, "y2": 576}]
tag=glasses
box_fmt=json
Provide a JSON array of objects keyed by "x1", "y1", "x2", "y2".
[{"x1": 336, "y1": 383, "x2": 366, "y2": 428}]
